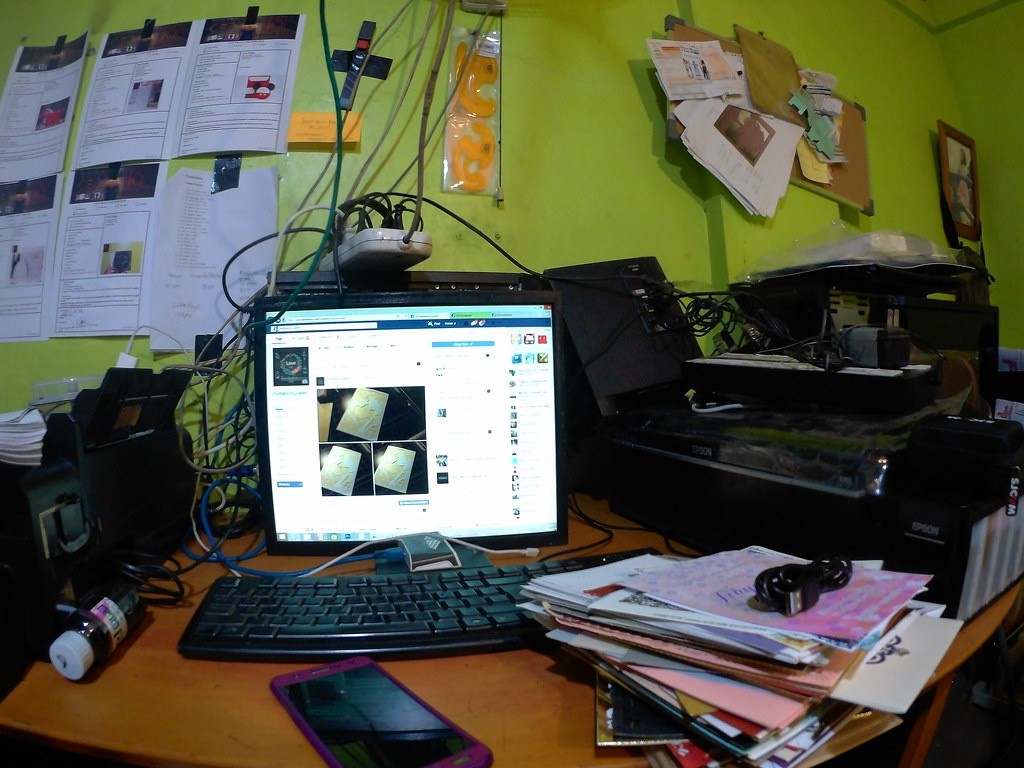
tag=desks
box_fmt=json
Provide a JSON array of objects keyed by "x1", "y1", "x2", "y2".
[{"x1": 0, "y1": 499, "x2": 908, "y2": 768}]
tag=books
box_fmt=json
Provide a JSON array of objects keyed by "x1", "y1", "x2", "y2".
[
  {"x1": 516, "y1": 545, "x2": 964, "y2": 768},
  {"x1": 0, "y1": 408, "x2": 47, "y2": 466}
]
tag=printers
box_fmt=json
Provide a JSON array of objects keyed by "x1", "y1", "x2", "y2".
[{"x1": 0, "y1": 364, "x2": 197, "y2": 587}]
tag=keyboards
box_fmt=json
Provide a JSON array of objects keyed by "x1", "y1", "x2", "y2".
[{"x1": 175, "y1": 546, "x2": 674, "y2": 665}]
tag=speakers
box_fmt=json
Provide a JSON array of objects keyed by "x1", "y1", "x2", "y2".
[
  {"x1": 540, "y1": 252, "x2": 706, "y2": 435},
  {"x1": 892, "y1": 414, "x2": 1022, "y2": 618}
]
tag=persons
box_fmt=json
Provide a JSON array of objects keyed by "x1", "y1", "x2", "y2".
[{"x1": 954, "y1": 149, "x2": 970, "y2": 224}]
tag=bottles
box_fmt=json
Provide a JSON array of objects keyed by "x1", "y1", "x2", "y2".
[{"x1": 49, "y1": 583, "x2": 146, "y2": 681}]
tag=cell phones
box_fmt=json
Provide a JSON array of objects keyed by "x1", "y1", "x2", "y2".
[{"x1": 269, "y1": 655, "x2": 494, "y2": 768}]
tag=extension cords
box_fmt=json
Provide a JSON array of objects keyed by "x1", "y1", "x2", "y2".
[{"x1": 320, "y1": 228, "x2": 433, "y2": 272}]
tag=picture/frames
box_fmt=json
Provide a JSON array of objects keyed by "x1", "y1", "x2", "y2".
[{"x1": 937, "y1": 118, "x2": 979, "y2": 243}]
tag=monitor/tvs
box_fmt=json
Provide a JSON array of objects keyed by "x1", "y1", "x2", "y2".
[{"x1": 244, "y1": 291, "x2": 571, "y2": 577}]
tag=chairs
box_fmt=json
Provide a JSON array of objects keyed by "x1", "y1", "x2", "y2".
[{"x1": 902, "y1": 578, "x2": 1024, "y2": 768}]
